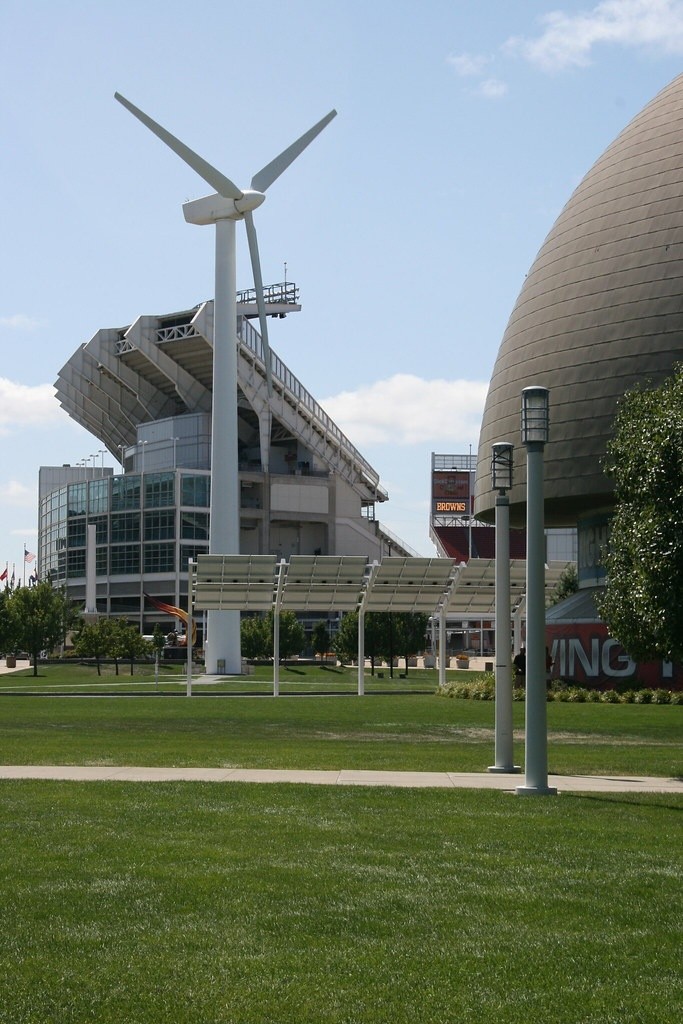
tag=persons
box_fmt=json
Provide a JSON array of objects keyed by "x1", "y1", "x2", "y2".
[
  {"x1": 513, "y1": 648, "x2": 526, "y2": 688},
  {"x1": 545, "y1": 646, "x2": 556, "y2": 688}
]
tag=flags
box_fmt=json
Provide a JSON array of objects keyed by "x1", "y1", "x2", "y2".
[
  {"x1": 10, "y1": 571, "x2": 15, "y2": 586},
  {"x1": 24, "y1": 550, "x2": 36, "y2": 563},
  {"x1": 0, "y1": 568, "x2": 7, "y2": 581}
]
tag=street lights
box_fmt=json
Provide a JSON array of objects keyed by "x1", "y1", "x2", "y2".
[
  {"x1": 518, "y1": 385, "x2": 561, "y2": 795},
  {"x1": 490, "y1": 439, "x2": 523, "y2": 774}
]
